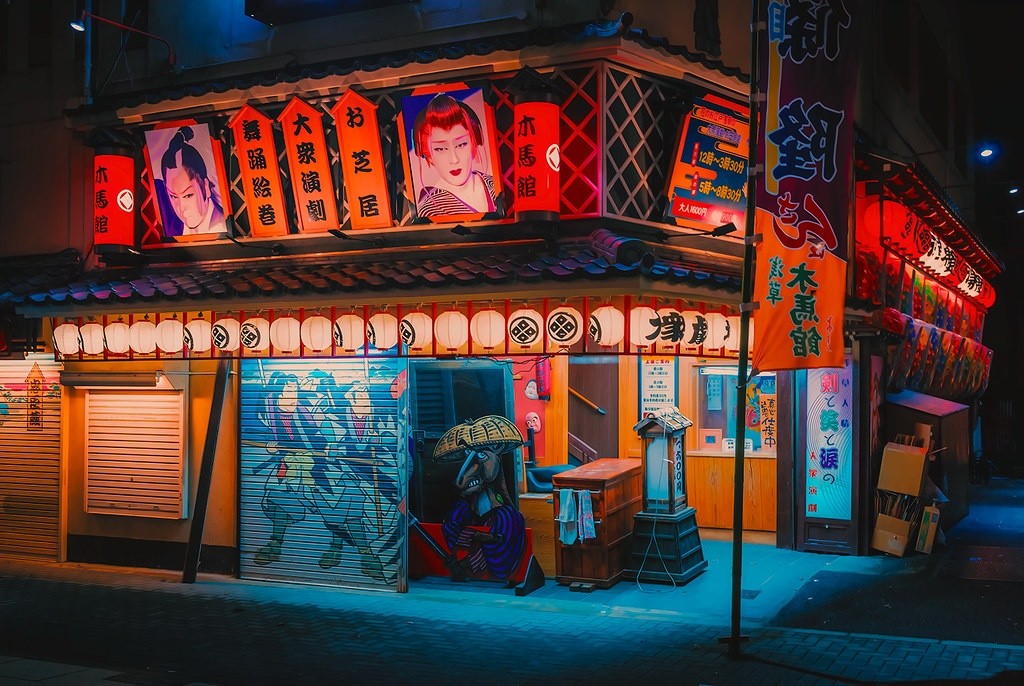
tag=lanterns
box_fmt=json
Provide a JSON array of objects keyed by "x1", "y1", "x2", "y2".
[
  {"x1": 864, "y1": 196, "x2": 996, "y2": 310},
  {"x1": 51, "y1": 303, "x2": 754, "y2": 358}
]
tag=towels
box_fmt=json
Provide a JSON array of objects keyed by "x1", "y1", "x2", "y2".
[
  {"x1": 577, "y1": 490, "x2": 597, "y2": 544},
  {"x1": 555, "y1": 488, "x2": 578, "y2": 546}
]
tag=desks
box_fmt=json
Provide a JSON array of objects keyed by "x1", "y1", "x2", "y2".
[{"x1": 551, "y1": 455, "x2": 642, "y2": 593}]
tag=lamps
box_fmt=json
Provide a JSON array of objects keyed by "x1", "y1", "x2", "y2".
[
  {"x1": 328, "y1": 227, "x2": 385, "y2": 248},
  {"x1": 655, "y1": 222, "x2": 737, "y2": 246},
  {"x1": 126, "y1": 248, "x2": 177, "y2": 260},
  {"x1": 946, "y1": 176, "x2": 1023, "y2": 195},
  {"x1": 450, "y1": 223, "x2": 483, "y2": 237},
  {"x1": 226, "y1": 234, "x2": 284, "y2": 255},
  {"x1": 68, "y1": 9, "x2": 177, "y2": 66},
  {"x1": 909, "y1": 142, "x2": 996, "y2": 161}
]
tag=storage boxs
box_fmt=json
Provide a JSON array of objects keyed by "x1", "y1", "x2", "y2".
[
  {"x1": 915, "y1": 502, "x2": 942, "y2": 555},
  {"x1": 876, "y1": 422, "x2": 933, "y2": 498},
  {"x1": 870, "y1": 502, "x2": 917, "y2": 558}
]
